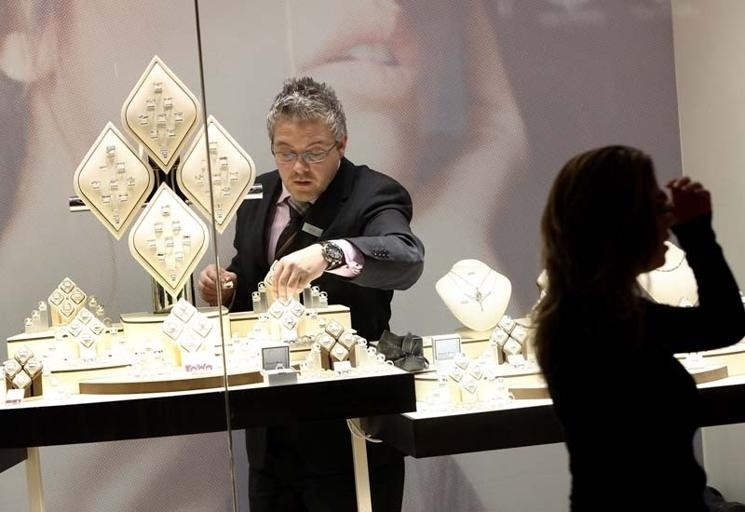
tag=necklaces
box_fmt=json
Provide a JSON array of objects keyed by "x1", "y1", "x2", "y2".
[
  {"x1": 448, "y1": 267, "x2": 498, "y2": 311},
  {"x1": 654, "y1": 251, "x2": 688, "y2": 273}
]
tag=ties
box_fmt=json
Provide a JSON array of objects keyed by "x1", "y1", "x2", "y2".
[{"x1": 275, "y1": 205, "x2": 305, "y2": 259}]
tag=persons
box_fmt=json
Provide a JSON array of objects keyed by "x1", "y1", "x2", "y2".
[
  {"x1": 529, "y1": 142, "x2": 745, "y2": 512},
  {"x1": 197, "y1": 76, "x2": 426, "y2": 512}
]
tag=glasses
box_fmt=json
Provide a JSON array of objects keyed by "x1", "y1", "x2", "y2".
[{"x1": 270, "y1": 140, "x2": 337, "y2": 166}]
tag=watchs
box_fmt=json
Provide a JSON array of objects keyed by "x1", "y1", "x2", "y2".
[{"x1": 316, "y1": 239, "x2": 345, "y2": 271}]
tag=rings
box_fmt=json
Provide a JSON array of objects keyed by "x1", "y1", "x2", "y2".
[
  {"x1": 247, "y1": 280, "x2": 395, "y2": 373},
  {"x1": 222, "y1": 280, "x2": 234, "y2": 290},
  {"x1": 447, "y1": 318, "x2": 525, "y2": 394}
]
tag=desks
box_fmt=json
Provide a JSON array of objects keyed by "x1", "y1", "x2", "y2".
[{"x1": 2, "y1": 364, "x2": 745, "y2": 512}]
